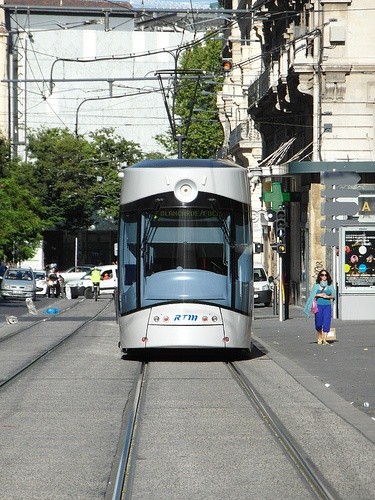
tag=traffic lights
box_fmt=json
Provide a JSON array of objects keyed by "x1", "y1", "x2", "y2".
[
  {"x1": 223, "y1": 60, "x2": 233, "y2": 73},
  {"x1": 276, "y1": 243, "x2": 287, "y2": 255},
  {"x1": 270, "y1": 242, "x2": 278, "y2": 252},
  {"x1": 275, "y1": 209, "x2": 286, "y2": 237}
]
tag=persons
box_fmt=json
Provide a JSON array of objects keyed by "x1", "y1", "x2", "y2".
[
  {"x1": 103, "y1": 273, "x2": 110, "y2": 280},
  {"x1": 23, "y1": 272, "x2": 28, "y2": 280},
  {"x1": 91, "y1": 266, "x2": 101, "y2": 293},
  {"x1": 303, "y1": 270, "x2": 336, "y2": 346}
]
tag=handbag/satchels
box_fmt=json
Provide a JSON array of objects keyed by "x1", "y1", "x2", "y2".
[{"x1": 310, "y1": 298, "x2": 318, "y2": 314}]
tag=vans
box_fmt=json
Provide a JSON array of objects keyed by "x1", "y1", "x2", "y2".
[{"x1": 252, "y1": 264, "x2": 275, "y2": 307}]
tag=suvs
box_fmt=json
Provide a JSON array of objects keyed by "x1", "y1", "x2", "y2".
[{"x1": 0, "y1": 266, "x2": 38, "y2": 301}]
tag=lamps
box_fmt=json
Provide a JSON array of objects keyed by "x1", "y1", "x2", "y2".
[{"x1": 219, "y1": 59, "x2": 232, "y2": 73}]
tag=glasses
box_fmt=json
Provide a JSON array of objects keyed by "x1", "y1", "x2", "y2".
[{"x1": 320, "y1": 274, "x2": 327, "y2": 276}]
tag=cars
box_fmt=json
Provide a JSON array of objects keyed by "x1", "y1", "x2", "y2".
[
  {"x1": 0, "y1": 264, "x2": 8, "y2": 284},
  {"x1": 53, "y1": 264, "x2": 119, "y2": 299},
  {"x1": 33, "y1": 270, "x2": 49, "y2": 299}
]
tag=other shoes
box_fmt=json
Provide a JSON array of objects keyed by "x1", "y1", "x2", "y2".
[
  {"x1": 92, "y1": 291, "x2": 94, "y2": 293},
  {"x1": 96, "y1": 291, "x2": 101, "y2": 295},
  {"x1": 318, "y1": 339, "x2": 329, "y2": 345}
]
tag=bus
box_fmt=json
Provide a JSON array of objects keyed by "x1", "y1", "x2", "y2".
[{"x1": 112, "y1": 68, "x2": 263, "y2": 357}]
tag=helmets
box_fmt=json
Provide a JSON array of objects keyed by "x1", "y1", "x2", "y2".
[{"x1": 49, "y1": 263, "x2": 57, "y2": 269}]
